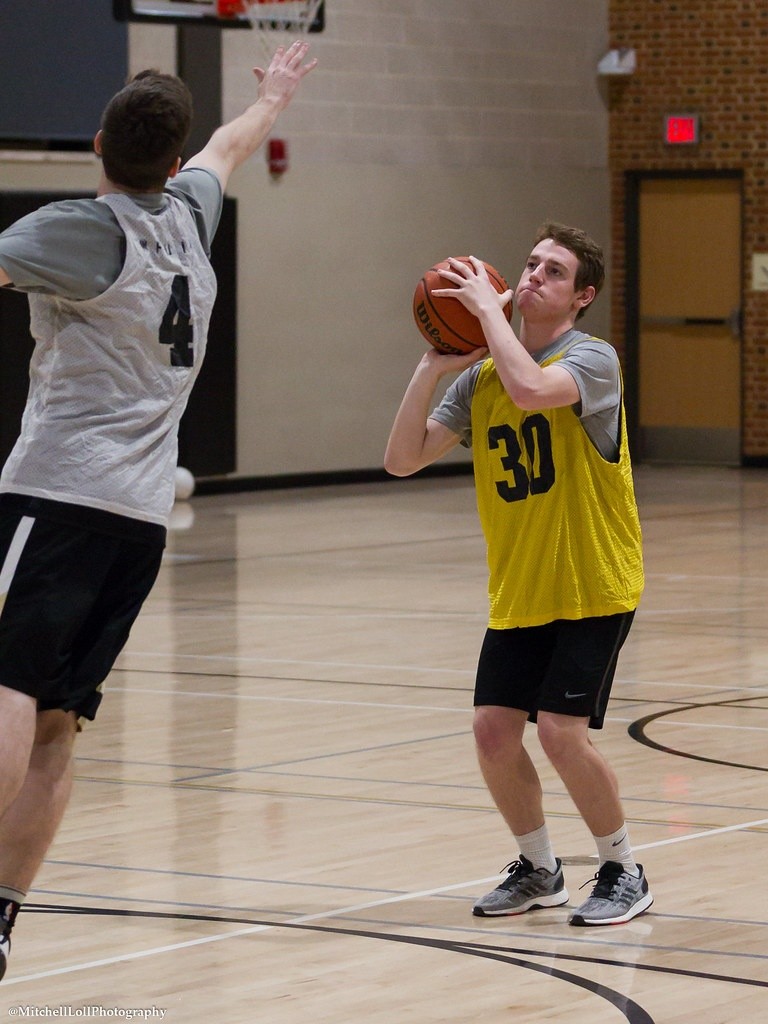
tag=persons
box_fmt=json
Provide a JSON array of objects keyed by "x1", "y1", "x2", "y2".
[
  {"x1": 0, "y1": 39, "x2": 323, "y2": 980},
  {"x1": 384, "y1": 222, "x2": 654, "y2": 926}
]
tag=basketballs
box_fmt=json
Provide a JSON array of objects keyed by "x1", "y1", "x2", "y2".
[{"x1": 413, "y1": 256, "x2": 515, "y2": 355}]
